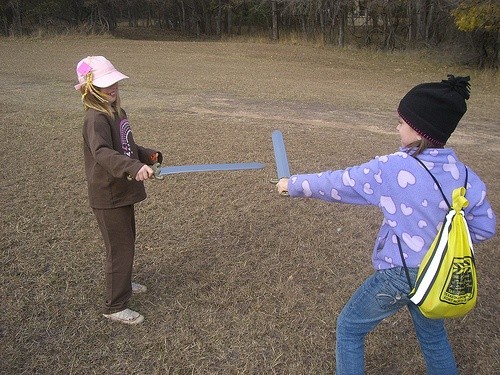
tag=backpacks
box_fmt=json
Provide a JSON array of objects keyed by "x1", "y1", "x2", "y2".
[{"x1": 392, "y1": 151, "x2": 478, "y2": 320}]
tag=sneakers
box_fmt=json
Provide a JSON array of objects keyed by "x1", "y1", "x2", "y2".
[
  {"x1": 129, "y1": 281, "x2": 147, "y2": 294},
  {"x1": 103, "y1": 306, "x2": 144, "y2": 325}
]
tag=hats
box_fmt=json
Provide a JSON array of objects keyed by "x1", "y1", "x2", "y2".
[
  {"x1": 75, "y1": 54, "x2": 129, "y2": 88},
  {"x1": 396, "y1": 72, "x2": 471, "y2": 147}
]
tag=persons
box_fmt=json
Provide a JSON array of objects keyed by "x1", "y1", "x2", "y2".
[
  {"x1": 73, "y1": 54, "x2": 165, "y2": 327},
  {"x1": 277, "y1": 72, "x2": 498, "y2": 375}
]
